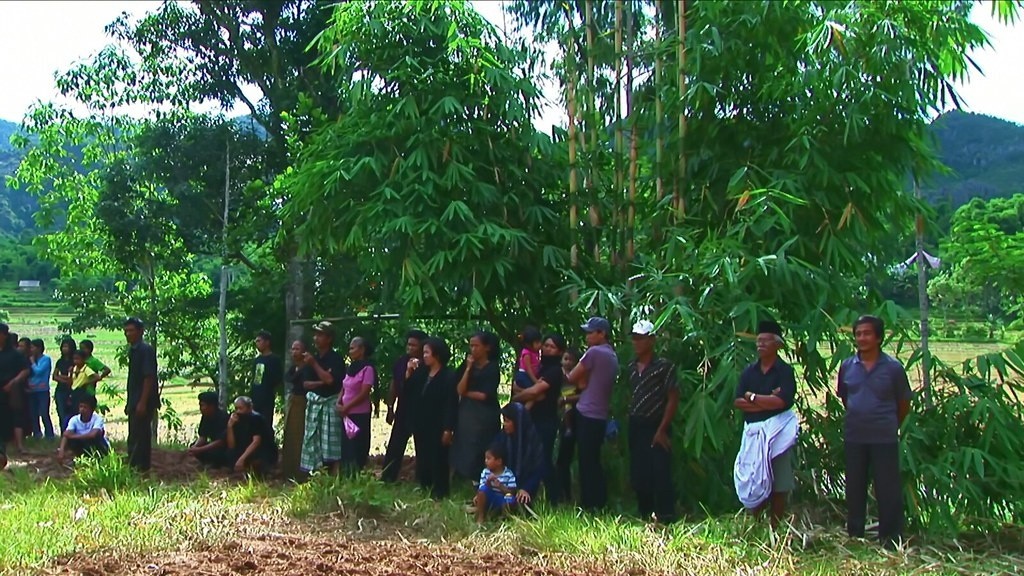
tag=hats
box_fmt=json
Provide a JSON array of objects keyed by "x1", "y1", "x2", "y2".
[
  {"x1": 632, "y1": 320, "x2": 654, "y2": 334},
  {"x1": 756, "y1": 321, "x2": 782, "y2": 337},
  {"x1": 580, "y1": 317, "x2": 610, "y2": 333},
  {"x1": 313, "y1": 321, "x2": 334, "y2": 334}
]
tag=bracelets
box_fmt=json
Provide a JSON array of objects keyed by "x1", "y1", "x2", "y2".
[{"x1": 749, "y1": 393, "x2": 755, "y2": 402}]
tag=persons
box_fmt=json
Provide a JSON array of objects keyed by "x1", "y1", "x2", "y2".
[
  {"x1": 124, "y1": 317, "x2": 161, "y2": 474},
  {"x1": 734, "y1": 322, "x2": 799, "y2": 532},
  {"x1": 627, "y1": 320, "x2": 678, "y2": 523},
  {"x1": 187, "y1": 391, "x2": 230, "y2": 466},
  {"x1": 281, "y1": 317, "x2": 620, "y2": 523},
  {"x1": 221, "y1": 396, "x2": 279, "y2": 481},
  {"x1": 0, "y1": 322, "x2": 113, "y2": 470},
  {"x1": 250, "y1": 330, "x2": 281, "y2": 424},
  {"x1": 837, "y1": 315, "x2": 911, "y2": 551}
]
